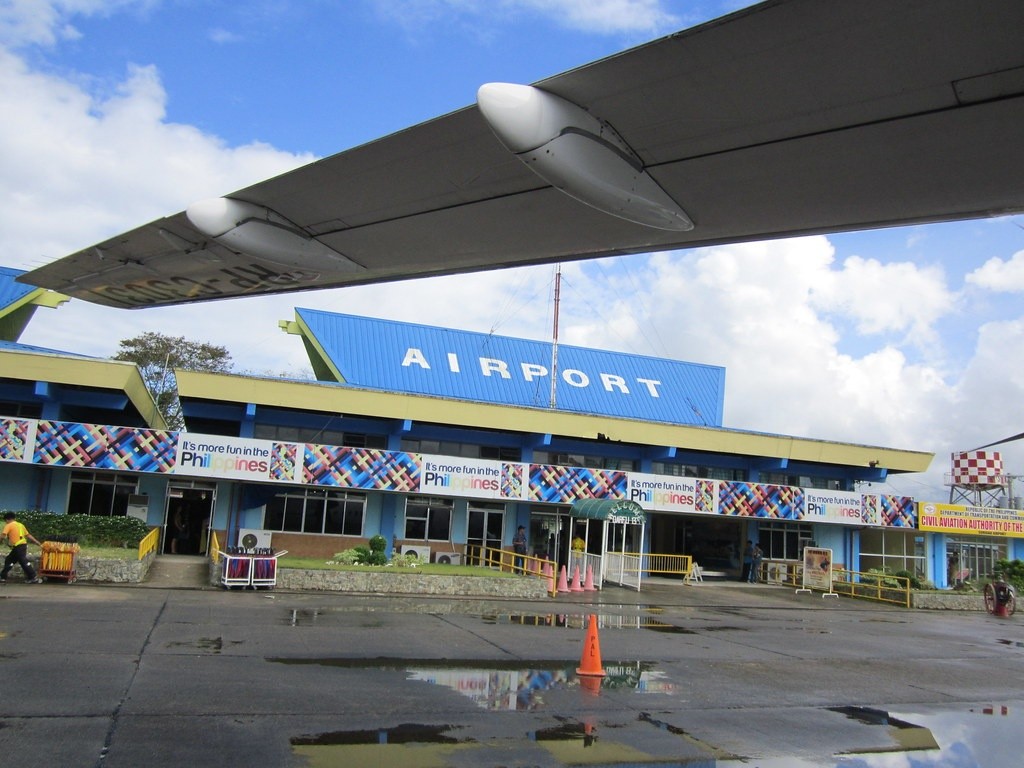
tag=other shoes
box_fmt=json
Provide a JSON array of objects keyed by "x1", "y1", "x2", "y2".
[
  {"x1": 24, "y1": 576, "x2": 38, "y2": 584},
  {"x1": 0, "y1": 577, "x2": 6, "y2": 582}
]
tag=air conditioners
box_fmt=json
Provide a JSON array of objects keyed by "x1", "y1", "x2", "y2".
[
  {"x1": 237, "y1": 528, "x2": 272, "y2": 554},
  {"x1": 767, "y1": 562, "x2": 787, "y2": 580},
  {"x1": 401, "y1": 545, "x2": 431, "y2": 563}
]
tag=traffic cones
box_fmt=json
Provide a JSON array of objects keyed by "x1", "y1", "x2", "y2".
[
  {"x1": 576, "y1": 614, "x2": 606, "y2": 676},
  {"x1": 583, "y1": 564, "x2": 597, "y2": 590},
  {"x1": 531, "y1": 554, "x2": 558, "y2": 593},
  {"x1": 557, "y1": 565, "x2": 571, "y2": 593},
  {"x1": 568, "y1": 565, "x2": 584, "y2": 591}
]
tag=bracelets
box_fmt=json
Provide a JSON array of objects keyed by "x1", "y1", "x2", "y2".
[{"x1": 35, "y1": 541, "x2": 41, "y2": 545}]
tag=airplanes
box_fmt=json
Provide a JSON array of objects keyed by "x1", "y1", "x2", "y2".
[{"x1": 16, "y1": 0, "x2": 1024, "y2": 309}]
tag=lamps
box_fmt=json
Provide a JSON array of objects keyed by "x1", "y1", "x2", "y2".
[{"x1": 435, "y1": 552, "x2": 461, "y2": 565}]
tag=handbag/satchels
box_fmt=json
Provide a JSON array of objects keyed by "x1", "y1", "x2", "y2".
[{"x1": 516, "y1": 544, "x2": 527, "y2": 554}]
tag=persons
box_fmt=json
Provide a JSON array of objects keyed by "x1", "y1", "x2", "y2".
[
  {"x1": 512, "y1": 525, "x2": 527, "y2": 575},
  {"x1": 0, "y1": 511, "x2": 42, "y2": 583},
  {"x1": 171, "y1": 505, "x2": 185, "y2": 554},
  {"x1": 572, "y1": 531, "x2": 585, "y2": 558},
  {"x1": 739, "y1": 540, "x2": 764, "y2": 582},
  {"x1": 549, "y1": 533, "x2": 555, "y2": 545},
  {"x1": 948, "y1": 552, "x2": 959, "y2": 587}
]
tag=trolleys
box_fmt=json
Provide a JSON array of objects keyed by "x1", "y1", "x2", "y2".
[{"x1": 38, "y1": 549, "x2": 77, "y2": 584}]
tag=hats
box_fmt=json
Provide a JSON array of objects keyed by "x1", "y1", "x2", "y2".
[{"x1": 518, "y1": 525, "x2": 526, "y2": 530}]
